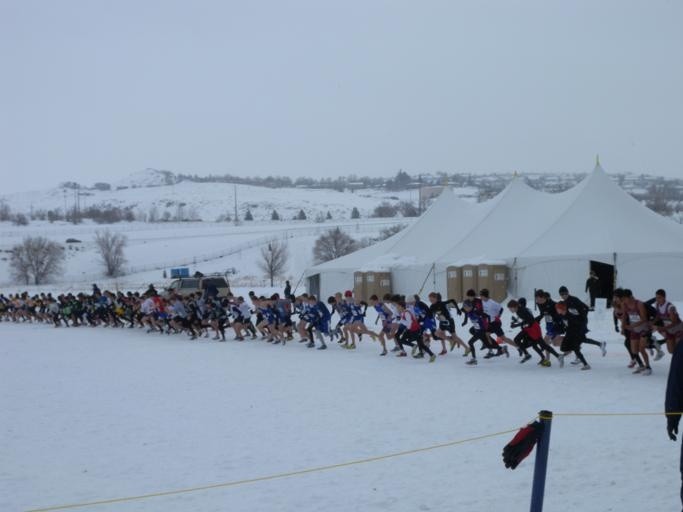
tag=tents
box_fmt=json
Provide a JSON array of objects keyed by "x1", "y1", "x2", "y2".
[{"x1": 305, "y1": 155, "x2": 683, "y2": 310}]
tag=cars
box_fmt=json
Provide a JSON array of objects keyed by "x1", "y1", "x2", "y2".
[{"x1": 65, "y1": 238, "x2": 81, "y2": 243}]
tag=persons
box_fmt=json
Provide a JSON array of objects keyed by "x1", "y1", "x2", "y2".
[
  {"x1": 2, "y1": 282, "x2": 297, "y2": 345},
  {"x1": 284, "y1": 280, "x2": 291, "y2": 297},
  {"x1": 611, "y1": 288, "x2": 683, "y2": 375},
  {"x1": 665, "y1": 338, "x2": 683, "y2": 512},
  {"x1": 456, "y1": 286, "x2": 607, "y2": 370},
  {"x1": 585, "y1": 271, "x2": 600, "y2": 310},
  {"x1": 291, "y1": 292, "x2": 470, "y2": 363}
]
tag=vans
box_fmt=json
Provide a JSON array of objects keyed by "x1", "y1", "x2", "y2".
[{"x1": 156, "y1": 275, "x2": 232, "y2": 312}]
